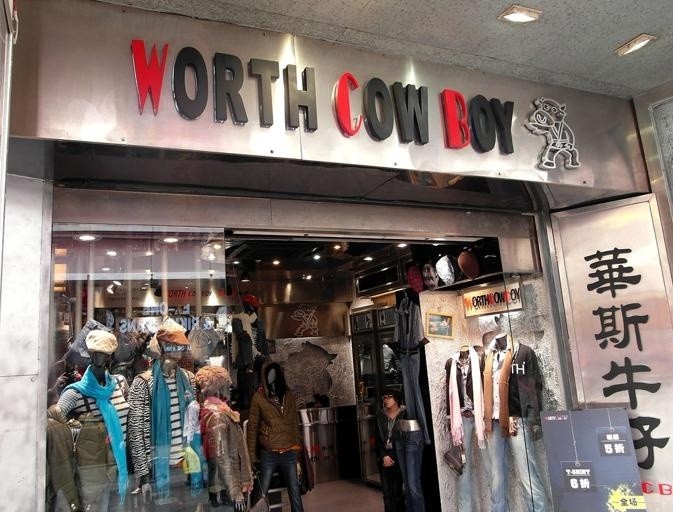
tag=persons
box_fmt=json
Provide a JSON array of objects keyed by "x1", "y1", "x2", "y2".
[
  {"x1": 480, "y1": 332, "x2": 546, "y2": 512},
  {"x1": 181, "y1": 388, "x2": 205, "y2": 488},
  {"x1": 247, "y1": 361, "x2": 305, "y2": 511},
  {"x1": 306, "y1": 393, "x2": 331, "y2": 408},
  {"x1": 128, "y1": 325, "x2": 204, "y2": 511},
  {"x1": 444, "y1": 344, "x2": 514, "y2": 512},
  {"x1": 393, "y1": 419, "x2": 426, "y2": 512},
  {"x1": 231, "y1": 292, "x2": 270, "y2": 411},
  {"x1": 48, "y1": 371, "x2": 82, "y2": 443},
  {"x1": 56, "y1": 330, "x2": 131, "y2": 511},
  {"x1": 374, "y1": 389, "x2": 406, "y2": 509},
  {"x1": 507, "y1": 413, "x2": 522, "y2": 439},
  {"x1": 194, "y1": 365, "x2": 255, "y2": 512}
]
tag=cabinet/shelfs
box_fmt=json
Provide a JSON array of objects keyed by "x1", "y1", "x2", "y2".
[{"x1": 348, "y1": 305, "x2": 406, "y2": 492}]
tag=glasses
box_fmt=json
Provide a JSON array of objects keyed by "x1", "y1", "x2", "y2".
[{"x1": 381, "y1": 394, "x2": 395, "y2": 401}]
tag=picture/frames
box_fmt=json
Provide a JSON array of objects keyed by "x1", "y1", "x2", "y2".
[{"x1": 426, "y1": 311, "x2": 455, "y2": 339}]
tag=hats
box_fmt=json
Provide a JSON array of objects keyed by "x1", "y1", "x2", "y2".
[
  {"x1": 422, "y1": 261, "x2": 439, "y2": 292},
  {"x1": 406, "y1": 264, "x2": 424, "y2": 292},
  {"x1": 435, "y1": 254, "x2": 455, "y2": 286},
  {"x1": 195, "y1": 365, "x2": 233, "y2": 397},
  {"x1": 84, "y1": 330, "x2": 120, "y2": 355},
  {"x1": 457, "y1": 250, "x2": 480, "y2": 281},
  {"x1": 156, "y1": 327, "x2": 189, "y2": 347}
]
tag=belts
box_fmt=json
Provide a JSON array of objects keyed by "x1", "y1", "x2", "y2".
[{"x1": 263, "y1": 444, "x2": 300, "y2": 454}]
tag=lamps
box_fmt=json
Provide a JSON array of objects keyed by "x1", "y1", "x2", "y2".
[
  {"x1": 143, "y1": 233, "x2": 162, "y2": 309},
  {"x1": 112, "y1": 281, "x2": 123, "y2": 286},
  {"x1": 104, "y1": 283, "x2": 117, "y2": 295}
]
